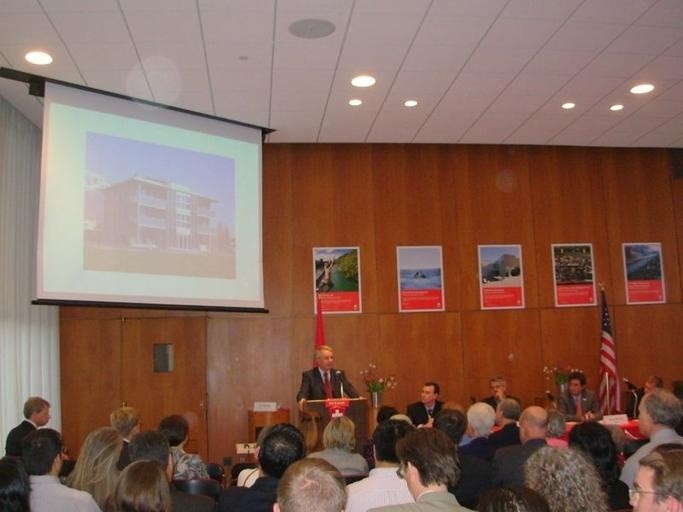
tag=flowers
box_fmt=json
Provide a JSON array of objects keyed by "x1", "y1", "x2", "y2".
[
  {"x1": 543, "y1": 365, "x2": 584, "y2": 385},
  {"x1": 360, "y1": 362, "x2": 399, "y2": 394}
]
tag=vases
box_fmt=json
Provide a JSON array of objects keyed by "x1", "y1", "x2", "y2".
[
  {"x1": 371, "y1": 391, "x2": 380, "y2": 408},
  {"x1": 560, "y1": 383, "x2": 567, "y2": 392}
]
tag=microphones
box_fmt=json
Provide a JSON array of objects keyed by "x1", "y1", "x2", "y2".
[
  {"x1": 469, "y1": 393, "x2": 476, "y2": 404},
  {"x1": 335, "y1": 369, "x2": 345, "y2": 383},
  {"x1": 546, "y1": 390, "x2": 555, "y2": 402}
]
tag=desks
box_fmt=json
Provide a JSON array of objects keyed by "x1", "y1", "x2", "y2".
[
  {"x1": 248, "y1": 407, "x2": 290, "y2": 449},
  {"x1": 490, "y1": 418, "x2": 649, "y2": 467}
]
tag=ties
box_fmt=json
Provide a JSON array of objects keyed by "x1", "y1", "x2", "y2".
[
  {"x1": 323, "y1": 371, "x2": 333, "y2": 399},
  {"x1": 428, "y1": 407, "x2": 434, "y2": 416},
  {"x1": 575, "y1": 396, "x2": 585, "y2": 418}
]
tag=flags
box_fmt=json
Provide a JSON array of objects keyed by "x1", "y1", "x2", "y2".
[
  {"x1": 598, "y1": 289, "x2": 620, "y2": 415},
  {"x1": 313, "y1": 294, "x2": 324, "y2": 367}
]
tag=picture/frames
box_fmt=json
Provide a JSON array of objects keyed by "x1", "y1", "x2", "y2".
[
  {"x1": 312, "y1": 247, "x2": 362, "y2": 315},
  {"x1": 477, "y1": 244, "x2": 525, "y2": 310},
  {"x1": 396, "y1": 245, "x2": 446, "y2": 313},
  {"x1": 622, "y1": 242, "x2": 666, "y2": 305},
  {"x1": 551, "y1": 243, "x2": 597, "y2": 307}
]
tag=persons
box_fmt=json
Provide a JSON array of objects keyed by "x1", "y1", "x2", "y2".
[
  {"x1": 316, "y1": 256, "x2": 335, "y2": 290},
  {"x1": 296, "y1": 345, "x2": 362, "y2": 411},
  {"x1": 0, "y1": 455, "x2": 32, "y2": 512},
  {"x1": 63, "y1": 390, "x2": 683, "y2": 512},
  {"x1": 404, "y1": 382, "x2": 443, "y2": 427},
  {"x1": 5, "y1": 396, "x2": 52, "y2": 456},
  {"x1": 21, "y1": 427, "x2": 103, "y2": 512},
  {"x1": 627, "y1": 375, "x2": 663, "y2": 421},
  {"x1": 480, "y1": 377, "x2": 521, "y2": 412},
  {"x1": 557, "y1": 372, "x2": 599, "y2": 422}
]
